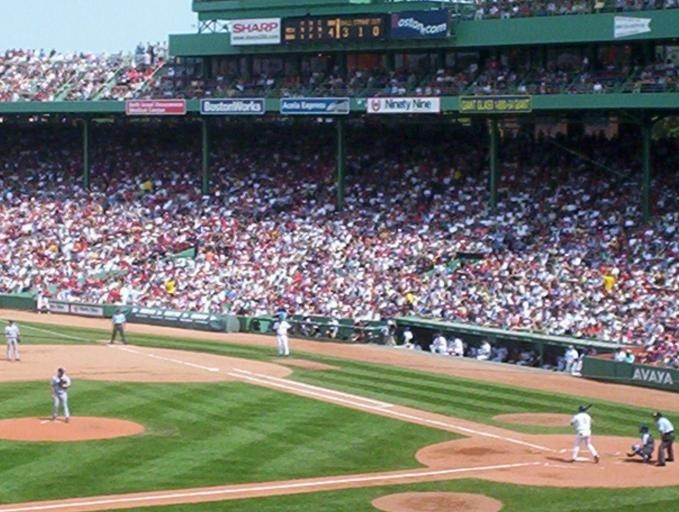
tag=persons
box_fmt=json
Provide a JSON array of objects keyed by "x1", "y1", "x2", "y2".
[
  {"x1": 50, "y1": 368, "x2": 71, "y2": 423},
  {"x1": 625, "y1": 423, "x2": 655, "y2": 464},
  {"x1": 0, "y1": 114, "x2": 678, "y2": 377},
  {"x1": 473, "y1": 0, "x2": 679, "y2": 22},
  {"x1": 1, "y1": 40, "x2": 678, "y2": 102},
  {"x1": 569, "y1": 405, "x2": 600, "y2": 463},
  {"x1": 651, "y1": 411, "x2": 675, "y2": 467}
]
